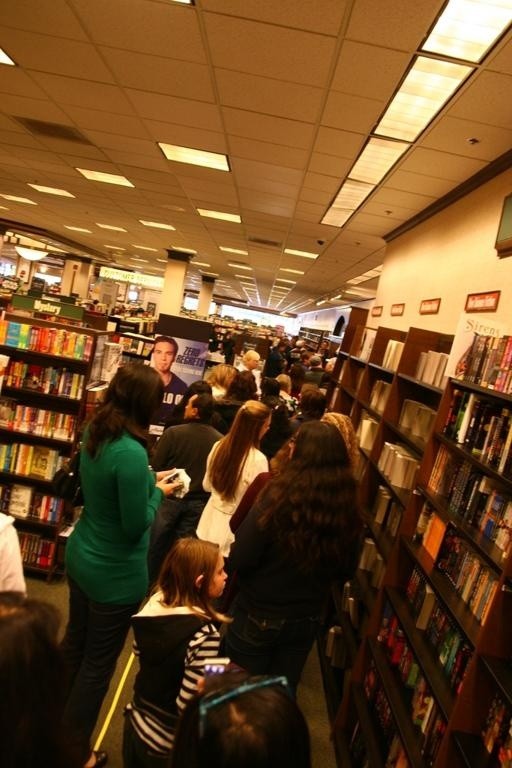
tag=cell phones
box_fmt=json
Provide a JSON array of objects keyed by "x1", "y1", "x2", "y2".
[{"x1": 202, "y1": 657, "x2": 229, "y2": 676}]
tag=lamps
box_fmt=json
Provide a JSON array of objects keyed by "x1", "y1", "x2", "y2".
[{"x1": 14, "y1": 245, "x2": 49, "y2": 262}]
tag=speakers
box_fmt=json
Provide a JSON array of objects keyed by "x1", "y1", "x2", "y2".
[{"x1": 333, "y1": 314, "x2": 345, "y2": 337}]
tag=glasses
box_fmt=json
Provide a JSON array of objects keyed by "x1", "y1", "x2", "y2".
[{"x1": 195, "y1": 672, "x2": 296, "y2": 743}]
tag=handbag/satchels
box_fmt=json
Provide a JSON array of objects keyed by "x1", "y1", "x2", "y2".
[{"x1": 49, "y1": 441, "x2": 87, "y2": 508}]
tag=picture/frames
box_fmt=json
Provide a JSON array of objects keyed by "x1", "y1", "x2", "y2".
[{"x1": 494, "y1": 192, "x2": 512, "y2": 259}]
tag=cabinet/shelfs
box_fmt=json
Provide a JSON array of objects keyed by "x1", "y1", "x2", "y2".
[
  {"x1": 317, "y1": 305, "x2": 512, "y2": 768},
  {"x1": 109, "y1": 307, "x2": 343, "y2": 398},
  {"x1": 2, "y1": 302, "x2": 108, "y2": 576}
]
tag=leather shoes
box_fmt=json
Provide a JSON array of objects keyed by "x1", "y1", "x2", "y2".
[{"x1": 93, "y1": 749, "x2": 109, "y2": 766}]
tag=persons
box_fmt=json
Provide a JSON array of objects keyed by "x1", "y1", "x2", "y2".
[
  {"x1": 123, "y1": 539, "x2": 230, "y2": 767},
  {"x1": 171, "y1": 673, "x2": 312, "y2": 768},
  {"x1": 0, "y1": 514, "x2": 28, "y2": 598},
  {"x1": 58, "y1": 365, "x2": 182, "y2": 768},
  {"x1": 1, "y1": 591, "x2": 86, "y2": 767}
]
